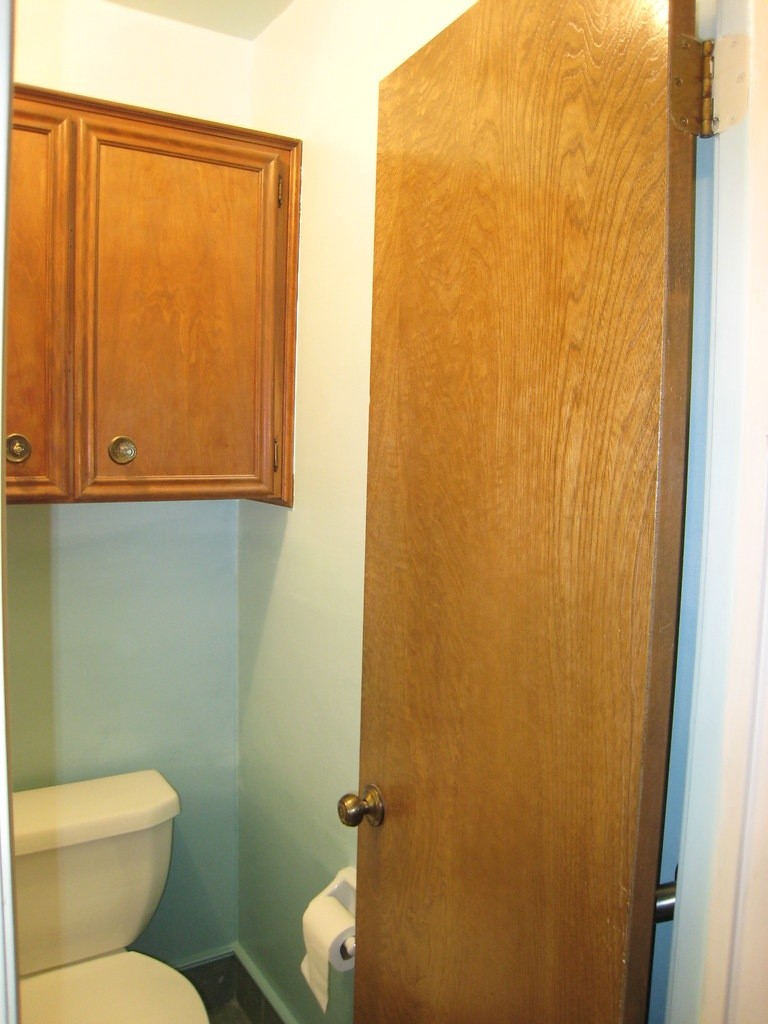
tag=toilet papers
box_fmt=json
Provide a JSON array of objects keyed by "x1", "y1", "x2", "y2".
[{"x1": 298, "y1": 893, "x2": 361, "y2": 1016}]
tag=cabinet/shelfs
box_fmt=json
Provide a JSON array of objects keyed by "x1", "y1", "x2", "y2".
[{"x1": 1, "y1": 81, "x2": 303, "y2": 508}]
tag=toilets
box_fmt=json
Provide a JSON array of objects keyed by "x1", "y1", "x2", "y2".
[{"x1": 13, "y1": 770, "x2": 216, "y2": 1024}]
